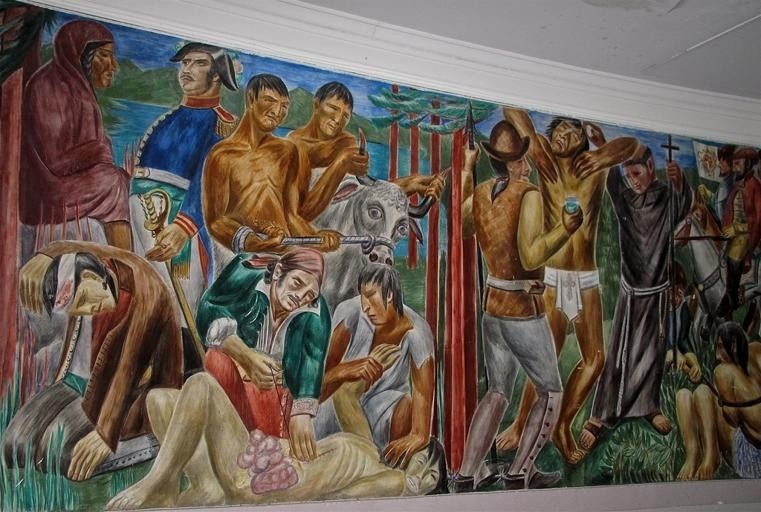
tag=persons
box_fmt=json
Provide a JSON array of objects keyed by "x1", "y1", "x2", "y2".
[{"x1": 5, "y1": 19, "x2": 761, "y2": 508}]
